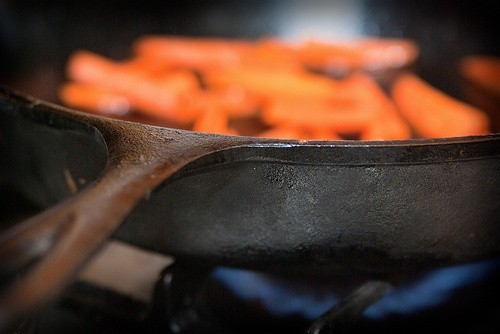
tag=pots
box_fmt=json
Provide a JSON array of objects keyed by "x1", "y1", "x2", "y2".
[{"x1": 0, "y1": 56, "x2": 499, "y2": 330}]
